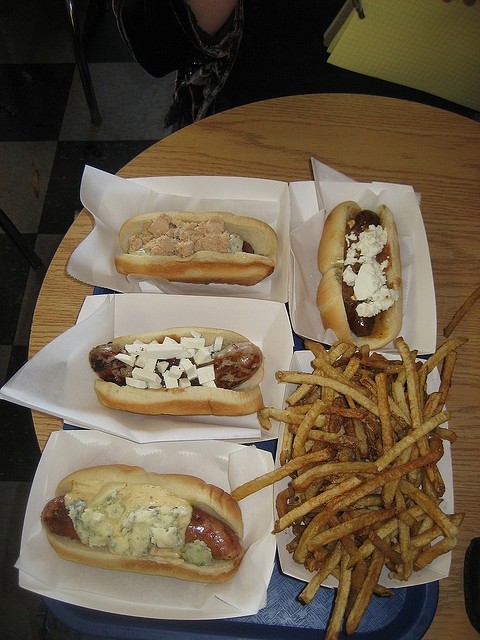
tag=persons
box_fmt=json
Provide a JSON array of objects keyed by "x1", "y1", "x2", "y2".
[{"x1": 106, "y1": 0, "x2": 476, "y2": 134}]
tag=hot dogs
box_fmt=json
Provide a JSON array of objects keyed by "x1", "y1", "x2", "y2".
[
  {"x1": 115, "y1": 211, "x2": 279, "y2": 285},
  {"x1": 41, "y1": 464, "x2": 247, "y2": 583},
  {"x1": 89, "y1": 325, "x2": 265, "y2": 415},
  {"x1": 317, "y1": 201, "x2": 403, "y2": 351}
]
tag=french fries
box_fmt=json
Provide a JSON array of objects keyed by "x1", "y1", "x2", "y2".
[{"x1": 234, "y1": 337, "x2": 472, "y2": 638}]
